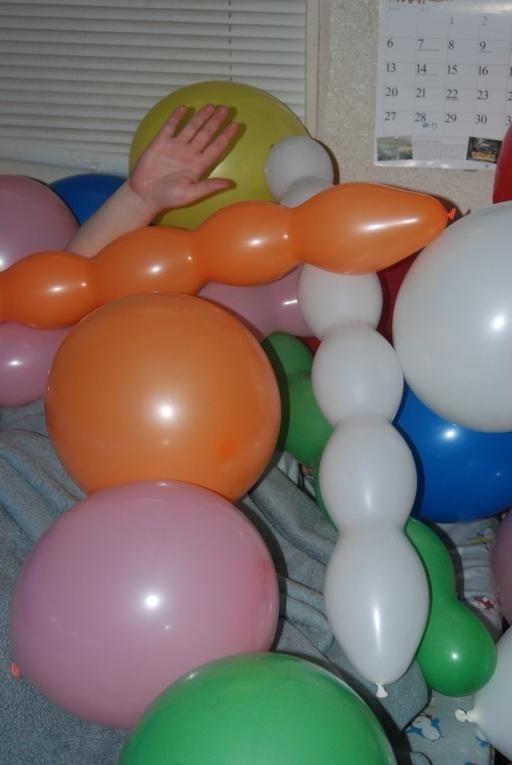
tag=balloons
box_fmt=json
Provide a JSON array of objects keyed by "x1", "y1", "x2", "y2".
[{"x1": 0, "y1": 77, "x2": 511, "y2": 764}]
